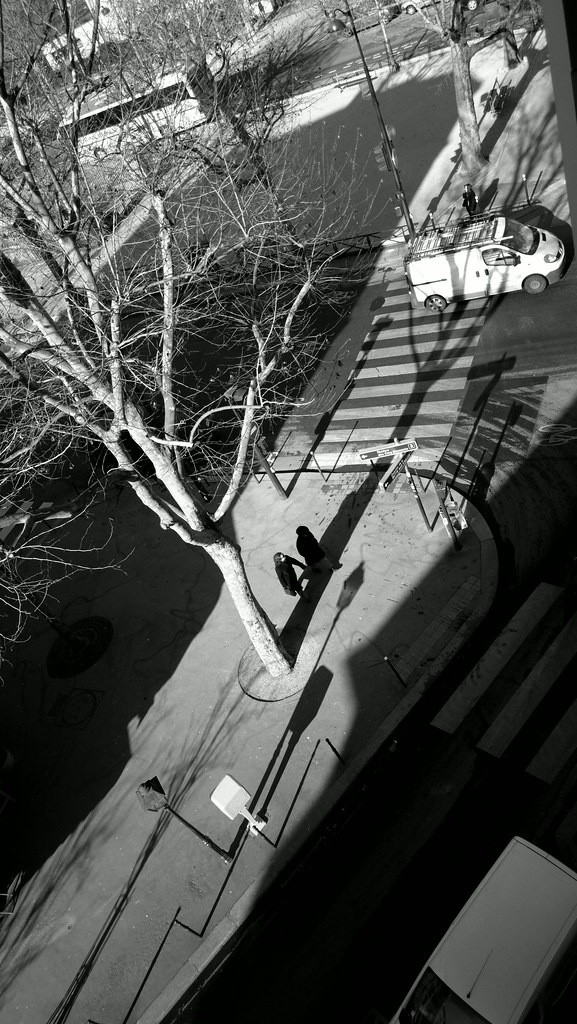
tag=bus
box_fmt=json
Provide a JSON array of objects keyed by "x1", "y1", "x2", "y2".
[{"x1": 58, "y1": 61, "x2": 217, "y2": 163}]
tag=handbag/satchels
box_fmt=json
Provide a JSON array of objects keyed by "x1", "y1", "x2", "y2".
[{"x1": 283, "y1": 586, "x2": 297, "y2": 596}]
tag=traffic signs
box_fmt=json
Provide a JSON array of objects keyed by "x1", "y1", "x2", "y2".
[
  {"x1": 356, "y1": 437, "x2": 420, "y2": 462},
  {"x1": 379, "y1": 448, "x2": 414, "y2": 493}
]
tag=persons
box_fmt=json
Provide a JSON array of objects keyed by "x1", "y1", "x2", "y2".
[
  {"x1": 296, "y1": 526, "x2": 343, "y2": 574},
  {"x1": 274, "y1": 552, "x2": 312, "y2": 599},
  {"x1": 462, "y1": 183, "x2": 476, "y2": 216}
]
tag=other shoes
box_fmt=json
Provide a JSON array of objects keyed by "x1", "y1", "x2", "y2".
[{"x1": 333, "y1": 564, "x2": 342, "y2": 569}]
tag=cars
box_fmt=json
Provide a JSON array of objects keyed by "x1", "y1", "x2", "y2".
[
  {"x1": 402, "y1": 0, "x2": 441, "y2": 15},
  {"x1": 463, "y1": 1, "x2": 497, "y2": 11},
  {"x1": 336, "y1": 1, "x2": 396, "y2": 39}
]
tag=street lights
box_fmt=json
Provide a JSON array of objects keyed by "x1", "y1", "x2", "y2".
[{"x1": 327, "y1": 0, "x2": 418, "y2": 232}]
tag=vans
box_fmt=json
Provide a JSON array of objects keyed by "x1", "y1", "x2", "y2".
[
  {"x1": 403, "y1": 216, "x2": 566, "y2": 313},
  {"x1": 391, "y1": 835, "x2": 577, "y2": 1024}
]
